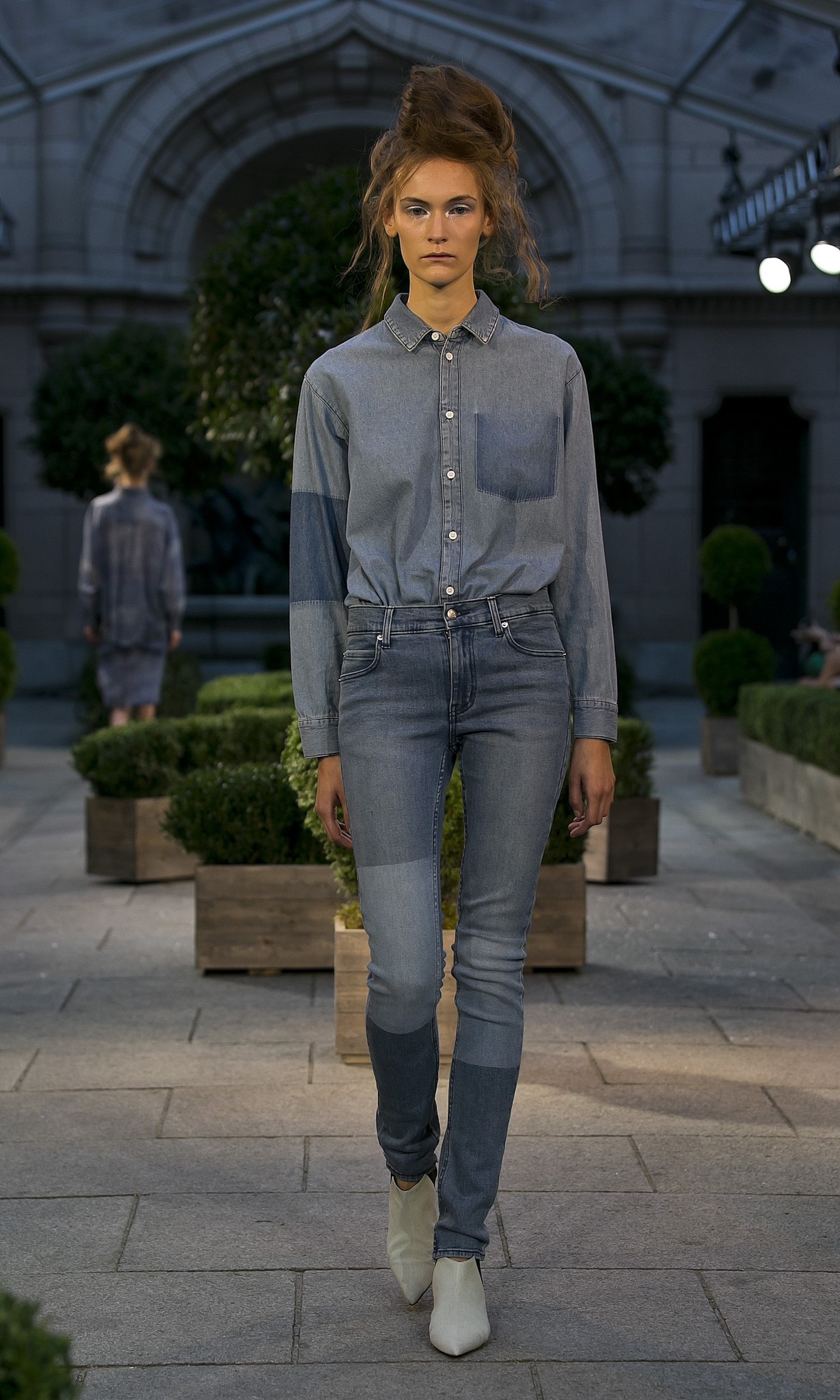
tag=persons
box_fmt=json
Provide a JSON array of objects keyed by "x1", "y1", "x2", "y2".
[
  {"x1": 77, "y1": 422, "x2": 187, "y2": 725},
  {"x1": 289, "y1": 61, "x2": 617, "y2": 1349}
]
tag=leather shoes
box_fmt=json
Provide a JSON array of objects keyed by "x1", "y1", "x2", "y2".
[
  {"x1": 388, "y1": 1163, "x2": 438, "y2": 1305},
  {"x1": 430, "y1": 1251, "x2": 491, "y2": 1356}
]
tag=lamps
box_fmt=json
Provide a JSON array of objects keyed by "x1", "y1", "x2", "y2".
[
  {"x1": 758, "y1": 222, "x2": 806, "y2": 293},
  {"x1": 810, "y1": 191, "x2": 840, "y2": 275}
]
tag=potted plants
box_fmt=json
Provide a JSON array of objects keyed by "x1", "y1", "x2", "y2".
[
  {"x1": 524, "y1": 776, "x2": 590, "y2": 968},
  {"x1": 282, "y1": 715, "x2": 465, "y2": 1068},
  {"x1": 582, "y1": 718, "x2": 659, "y2": 882},
  {"x1": 737, "y1": 682, "x2": 840, "y2": 850},
  {"x1": 160, "y1": 761, "x2": 336, "y2": 970},
  {"x1": 73, "y1": 706, "x2": 280, "y2": 882},
  {"x1": 692, "y1": 526, "x2": 773, "y2": 774}
]
